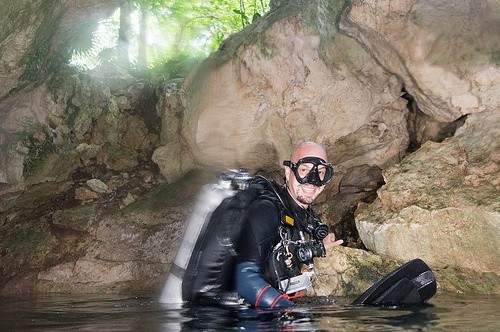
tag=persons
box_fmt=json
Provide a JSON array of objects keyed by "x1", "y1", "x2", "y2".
[{"x1": 182, "y1": 141, "x2": 344, "y2": 309}]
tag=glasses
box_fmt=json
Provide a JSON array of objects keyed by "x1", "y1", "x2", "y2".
[{"x1": 283, "y1": 157, "x2": 333, "y2": 187}]
